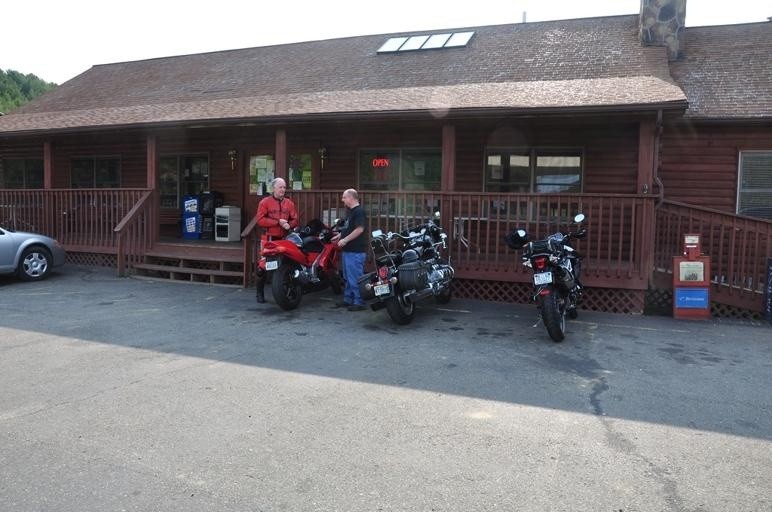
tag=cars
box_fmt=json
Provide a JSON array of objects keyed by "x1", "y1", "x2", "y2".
[{"x1": 0, "y1": 225, "x2": 68, "y2": 282}]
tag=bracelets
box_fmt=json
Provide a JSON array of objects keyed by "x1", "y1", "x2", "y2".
[{"x1": 343, "y1": 239, "x2": 348, "y2": 244}]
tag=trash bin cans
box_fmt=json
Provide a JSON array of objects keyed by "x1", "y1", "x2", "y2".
[
  {"x1": 214, "y1": 205, "x2": 242, "y2": 243},
  {"x1": 765, "y1": 257, "x2": 772, "y2": 324},
  {"x1": 671, "y1": 233, "x2": 712, "y2": 321}
]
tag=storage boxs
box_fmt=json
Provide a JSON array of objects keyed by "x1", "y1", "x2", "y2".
[{"x1": 180, "y1": 193, "x2": 224, "y2": 242}]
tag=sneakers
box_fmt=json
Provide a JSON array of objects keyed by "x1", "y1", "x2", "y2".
[
  {"x1": 256, "y1": 292, "x2": 264, "y2": 303},
  {"x1": 336, "y1": 301, "x2": 365, "y2": 311}
]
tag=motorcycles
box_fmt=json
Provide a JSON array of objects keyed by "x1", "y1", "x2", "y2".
[
  {"x1": 356, "y1": 211, "x2": 455, "y2": 325},
  {"x1": 505, "y1": 213, "x2": 588, "y2": 343},
  {"x1": 257, "y1": 219, "x2": 344, "y2": 311}
]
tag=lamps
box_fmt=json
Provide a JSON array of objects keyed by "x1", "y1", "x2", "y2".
[
  {"x1": 224, "y1": 147, "x2": 238, "y2": 171},
  {"x1": 317, "y1": 147, "x2": 329, "y2": 171}
]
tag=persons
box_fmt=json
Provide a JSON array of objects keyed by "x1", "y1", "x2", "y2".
[
  {"x1": 255, "y1": 178, "x2": 298, "y2": 303},
  {"x1": 331, "y1": 189, "x2": 368, "y2": 311}
]
